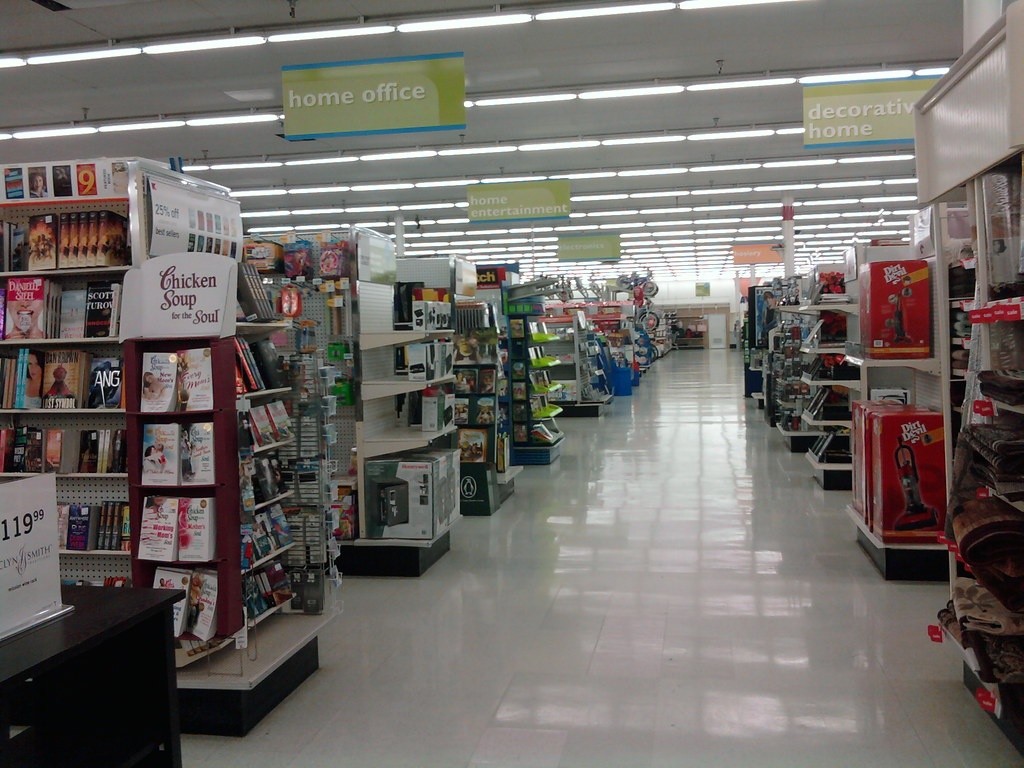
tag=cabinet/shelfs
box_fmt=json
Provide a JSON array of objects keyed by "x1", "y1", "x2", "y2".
[
  {"x1": 674, "y1": 338, "x2": 704, "y2": 348},
  {"x1": 355, "y1": 328, "x2": 463, "y2": 548},
  {"x1": 843, "y1": 354, "x2": 950, "y2": 583},
  {"x1": 775, "y1": 304, "x2": 859, "y2": 491},
  {"x1": 938, "y1": 297, "x2": 1024, "y2": 756},
  {"x1": 507, "y1": 305, "x2": 566, "y2": 465},
  {"x1": 573, "y1": 315, "x2": 613, "y2": 417},
  {"x1": 0, "y1": 156, "x2": 343, "y2": 768}
]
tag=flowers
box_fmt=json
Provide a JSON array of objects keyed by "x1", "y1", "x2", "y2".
[{"x1": 819, "y1": 272, "x2": 845, "y2": 294}]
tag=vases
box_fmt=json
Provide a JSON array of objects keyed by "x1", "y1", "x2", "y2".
[{"x1": 819, "y1": 293, "x2": 851, "y2": 304}]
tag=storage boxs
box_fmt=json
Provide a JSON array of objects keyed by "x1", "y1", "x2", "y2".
[
  {"x1": 851, "y1": 259, "x2": 948, "y2": 545},
  {"x1": 362, "y1": 301, "x2": 460, "y2": 539}
]
